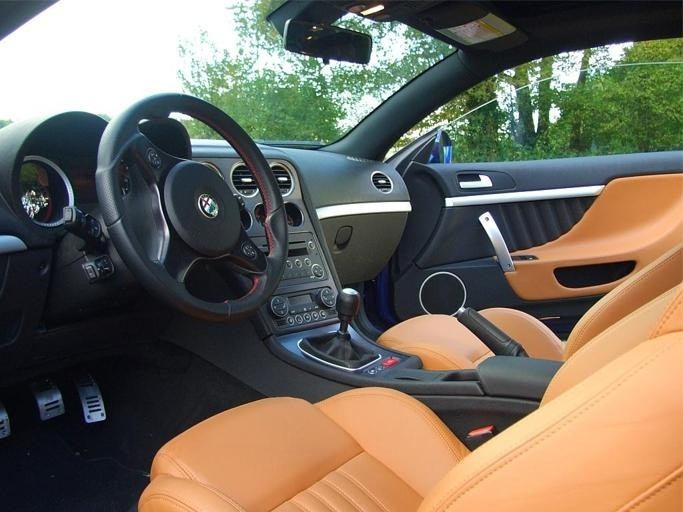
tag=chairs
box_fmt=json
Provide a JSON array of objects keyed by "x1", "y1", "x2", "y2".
[
  {"x1": 372, "y1": 233, "x2": 683, "y2": 374},
  {"x1": 137, "y1": 283, "x2": 682, "y2": 512}
]
